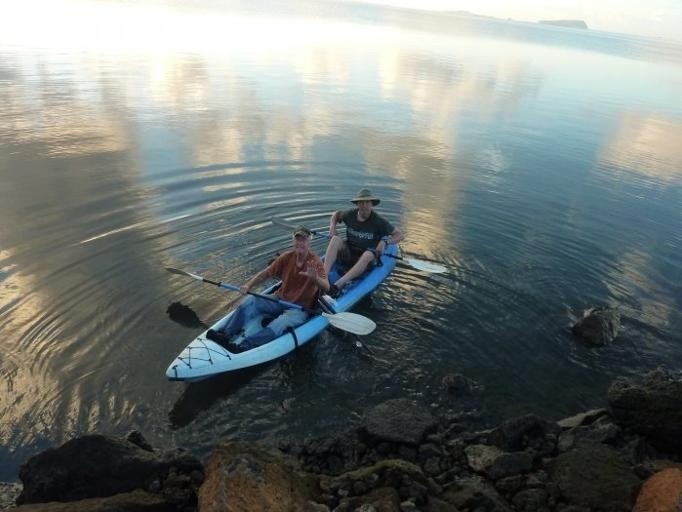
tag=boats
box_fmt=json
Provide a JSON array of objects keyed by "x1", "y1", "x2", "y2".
[{"x1": 165, "y1": 237, "x2": 398, "y2": 381}]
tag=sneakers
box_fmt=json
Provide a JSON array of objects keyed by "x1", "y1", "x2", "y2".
[
  {"x1": 328, "y1": 285, "x2": 340, "y2": 297},
  {"x1": 207, "y1": 329, "x2": 240, "y2": 353}
]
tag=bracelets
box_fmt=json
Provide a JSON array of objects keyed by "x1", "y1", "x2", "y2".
[{"x1": 382, "y1": 236, "x2": 389, "y2": 247}]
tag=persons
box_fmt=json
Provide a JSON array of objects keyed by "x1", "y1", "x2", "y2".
[
  {"x1": 204, "y1": 224, "x2": 331, "y2": 354},
  {"x1": 322, "y1": 188, "x2": 406, "y2": 298}
]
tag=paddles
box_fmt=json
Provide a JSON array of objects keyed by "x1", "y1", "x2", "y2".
[
  {"x1": 165, "y1": 266, "x2": 377, "y2": 336},
  {"x1": 272, "y1": 216, "x2": 447, "y2": 275}
]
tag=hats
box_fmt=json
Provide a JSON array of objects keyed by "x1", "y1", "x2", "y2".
[
  {"x1": 292, "y1": 224, "x2": 312, "y2": 239},
  {"x1": 349, "y1": 188, "x2": 380, "y2": 207}
]
